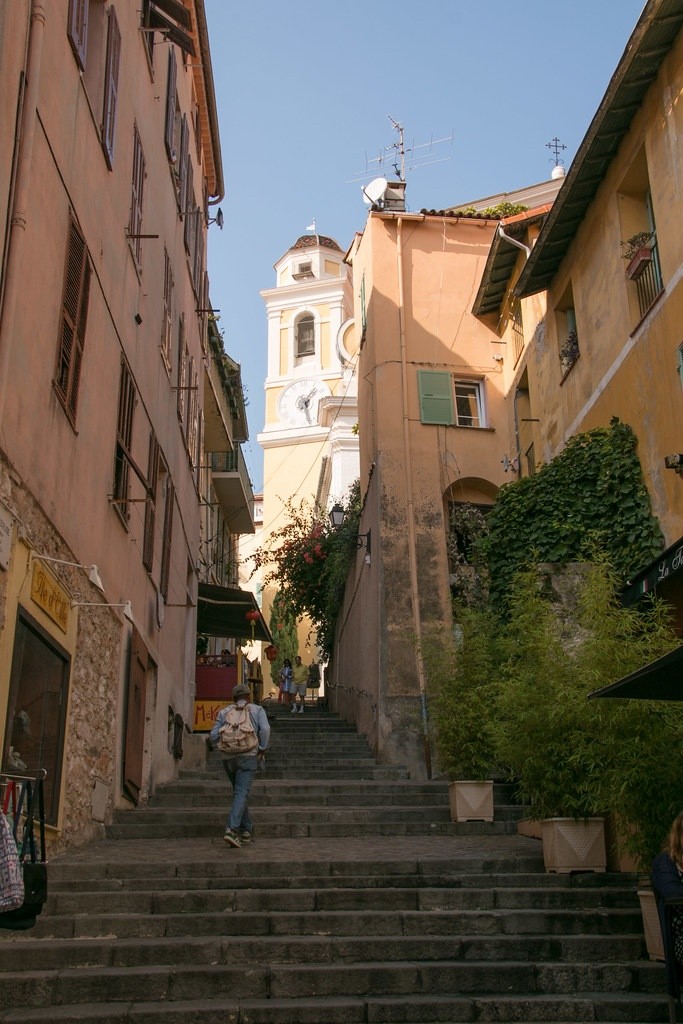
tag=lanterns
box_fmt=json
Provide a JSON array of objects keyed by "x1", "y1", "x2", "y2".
[
  {"x1": 263, "y1": 645, "x2": 280, "y2": 662},
  {"x1": 246, "y1": 608, "x2": 260, "y2": 639}
]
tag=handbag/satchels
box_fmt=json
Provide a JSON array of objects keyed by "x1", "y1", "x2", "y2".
[{"x1": 0, "y1": 777, "x2": 47, "y2": 930}]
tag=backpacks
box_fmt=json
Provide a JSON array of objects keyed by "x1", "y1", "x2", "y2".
[{"x1": 220, "y1": 701, "x2": 260, "y2": 752}]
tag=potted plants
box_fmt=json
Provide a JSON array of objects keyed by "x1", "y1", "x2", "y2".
[
  {"x1": 623, "y1": 231, "x2": 655, "y2": 280},
  {"x1": 489, "y1": 600, "x2": 659, "y2": 876},
  {"x1": 393, "y1": 577, "x2": 540, "y2": 823}
]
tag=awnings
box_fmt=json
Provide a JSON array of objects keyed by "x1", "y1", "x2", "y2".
[
  {"x1": 197, "y1": 581, "x2": 275, "y2": 645},
  {"x1": 587, "y1": 643, "x2": 683, "y2": 700},
  {"x1": 615, "y1": 536, "x2": 683, "y2": 613}
]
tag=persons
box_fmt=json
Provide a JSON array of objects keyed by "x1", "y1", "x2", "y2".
[
  {"x1": 209, "y1": 683, "x2": 271, "y2": 848},
  {"x1": 307, "y1": 661, "x2": 320, "y2": 689},
  {"x1": 220, "y1": 649, "x2": 237, "y2": 670},
  {"x1": 278, "y1": 656, "x2": 310, "y2": 714},
  {"x1": 651, "y1": 811, "x2": 683, "y2": 1004}
]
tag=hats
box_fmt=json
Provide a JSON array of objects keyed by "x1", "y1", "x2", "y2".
[{"x1": 232, "y1": 684, "x2": 249, "y2": 696}]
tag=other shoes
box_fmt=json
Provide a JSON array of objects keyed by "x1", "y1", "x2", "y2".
[
  {"x1": 298, "y1": 707, "x2": 304, "y2": 714},
  {"x1": 291, "y1": 709, "x2": 298, "y2": 714},
  {"x1": 224, "y1": 829, "x2": 241, "y2": 847},
  {"x1": 242, "y1": 833, "x2": 256, "y2": 843}
]
